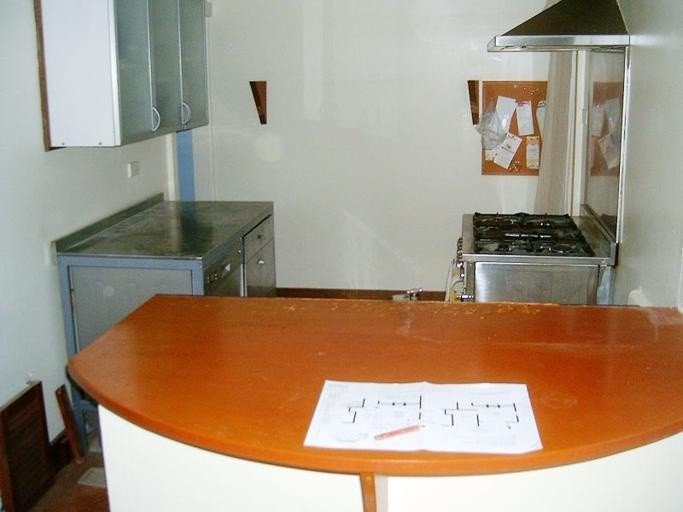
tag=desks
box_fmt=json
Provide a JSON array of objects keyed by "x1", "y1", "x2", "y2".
[{"x1": 64, "y1": 292, "x2": 682, "y2": 511}]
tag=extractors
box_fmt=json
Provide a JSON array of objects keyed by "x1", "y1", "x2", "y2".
[{"x1": 485, "y1": 0, "x2": 629, "y2": 53}]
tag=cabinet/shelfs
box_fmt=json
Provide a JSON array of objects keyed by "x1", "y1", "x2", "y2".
[
  {"x1": 61, "y1": 210, "x2": 278, "y2": 457},
  {"x1": 35, "y1": 0, "x2": 212, "y2": 149}
]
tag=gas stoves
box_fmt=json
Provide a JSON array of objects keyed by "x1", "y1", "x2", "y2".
[{"x1": 473, "y1": 212, "x2": 596, "y2": 259}]
